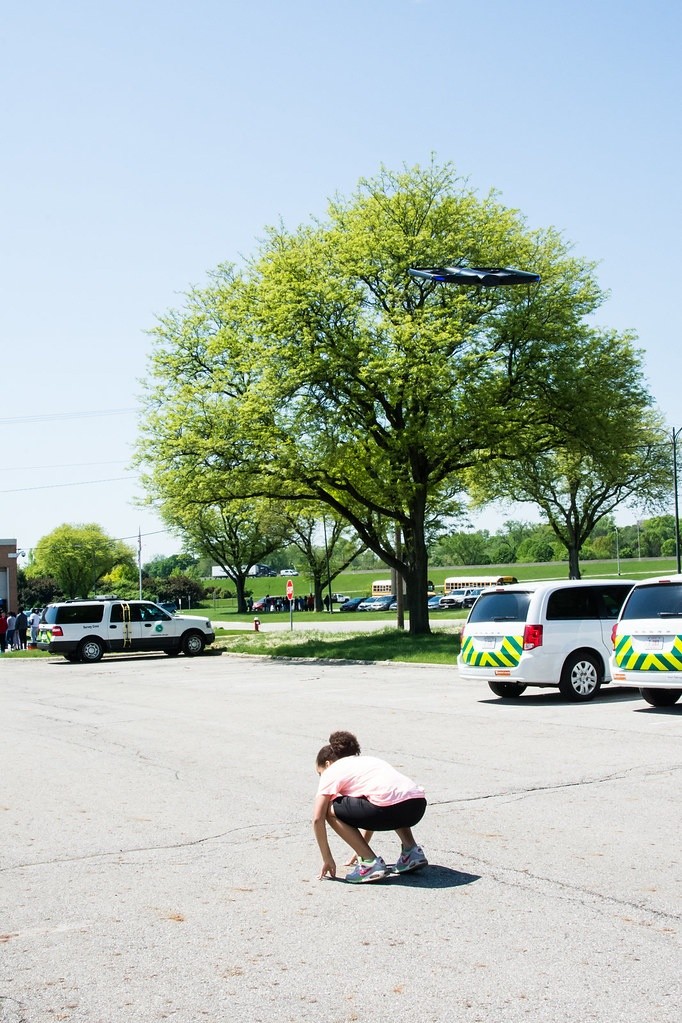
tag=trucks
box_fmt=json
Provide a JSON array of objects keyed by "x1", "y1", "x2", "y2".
[{"x1": 212, "y1": 564, "x2": 277, "y2": 578}]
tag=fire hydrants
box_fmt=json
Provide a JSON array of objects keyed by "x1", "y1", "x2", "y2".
[{"x1": 254, "y1": 617, "x2": 261, "y2": 631}]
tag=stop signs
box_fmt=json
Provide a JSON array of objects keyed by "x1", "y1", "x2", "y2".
[{"x1": 286, "y1": 581, "x2": 293, "y2": 600}]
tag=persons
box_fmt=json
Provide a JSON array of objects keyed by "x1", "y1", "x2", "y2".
[
  {"x1": 262, "y1": 594, "x2": 290, "y2": 612},
  {"x1": 247, "y1": 597, "x2": 253, "y2": 612},
  {"x1": 0, "y1": 606, "x2": 27, "y2": 653},
  {"x1": 29, "y1": 609, "x2": 41, "y2": 642},
  {"x1": 313, "y1": 731, "x2": 428, "y2": 884},
  {"x1": 324, "y1": 595, "x2": 330, "y2": 611},
  {"x1": 294, "y1": 593, "x2": 314, "y2": 611}
]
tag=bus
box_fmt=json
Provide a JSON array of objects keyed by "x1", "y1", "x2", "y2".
[
  {"x1": 444, "y1": 576, "x2": 520, "y2": 595},
  {"x1": 372, "y1": 580, "x2": 436, "y2": 597}
]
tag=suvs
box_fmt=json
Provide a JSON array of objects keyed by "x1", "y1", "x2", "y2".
[{"x1": 28, "y1": 595, "x2": 215, "y2": 664}]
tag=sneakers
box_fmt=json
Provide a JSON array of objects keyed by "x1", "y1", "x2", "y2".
[
  {"x1": 391, "y1": 844, "x2": 428, "y2": 874},
  {"x1": 345, "y1": 855, "x2": 389, "y2": 883}
]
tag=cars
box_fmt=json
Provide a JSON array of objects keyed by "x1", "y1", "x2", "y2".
[
  {"x1": 428, "y1": 596, "x2": 443, "y2": 609},
  {"x1": 608, "y1": 574, "x2": 682, "y2": 706},
  {"x1": 371, "y1": 595, "x2": 397, "y2": 611},
  {"x1": 340, "y1": 597, "x2": 368, "y2": 612},
  {"x1": 439, "y1": 588, "x2": 474, "y2": 608},
  {"x1": 462, "y1": 588, "x2": 485, "y2": 608},
  {"x1": 357, "y1": 596, "x2": 381, "y2": 612},
  {"x1": 457, "y1": 579, "x2": 639, "y2": 702},
  {"x1": 280, "y1": 570, "x2": 299, "y2": 576},
  {"x1": 152, "y1": 602, "x2": 177, "y2": 614},
  {"x1": 248, "y1": 596, "x2": 296, "y2": 612},
  {"x1": 389, "y1": 602, "x2": 398, "y2": 611}
]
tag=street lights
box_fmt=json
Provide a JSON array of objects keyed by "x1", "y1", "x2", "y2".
[{"x1": 615, "y1": 527, "x2": 620, "y2": 575}]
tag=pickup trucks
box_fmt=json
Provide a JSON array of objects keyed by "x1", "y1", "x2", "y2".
[{"x1": 322, "y1": 594, "x2": 349, "y2": 603}]
